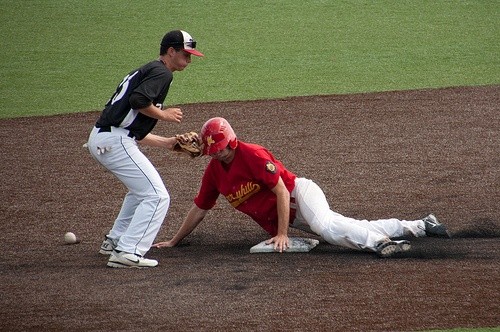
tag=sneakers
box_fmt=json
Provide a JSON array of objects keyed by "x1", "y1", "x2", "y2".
[
  {"x1": 107, "y1": 246, "x2": 158, "y2": 268},
  {"x1": 376, "y1": 239, "x2": 412, "y2": 258},
  {"x1": 99, "y1": 235, "x2": 115, "y2": 254},
  {"x1": 422, "y1": 213, "x2": 451, "y2": 239}
]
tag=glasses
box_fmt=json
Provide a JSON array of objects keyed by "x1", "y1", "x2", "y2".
[{"x1": 171, "y1": 41, "x2": 197, "y2": 48}]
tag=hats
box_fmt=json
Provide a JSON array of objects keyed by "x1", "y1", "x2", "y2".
[{"x1": 162, "y1": 29, "x2": 203, "y2": 57}]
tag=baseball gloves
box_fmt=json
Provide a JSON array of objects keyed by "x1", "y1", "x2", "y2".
[{"x1": 173, "y1": 133, "x2": 202, "y2": 158}]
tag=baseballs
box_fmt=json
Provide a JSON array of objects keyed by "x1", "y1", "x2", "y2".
[{"x1": 64, "y1": 233, "x2": 75, "y2": 245}]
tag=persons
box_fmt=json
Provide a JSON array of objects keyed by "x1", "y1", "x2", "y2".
[
  {"x1": 151, "y1": 117, "x2": 452, "y2": 257},
  {"x1": 88, "y1": 30, "x2": 204, "y2": 268}
]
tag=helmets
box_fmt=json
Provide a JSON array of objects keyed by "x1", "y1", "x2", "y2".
[{"x1": 201, "y1": 117, "x2": 238, "y2": 155}]
